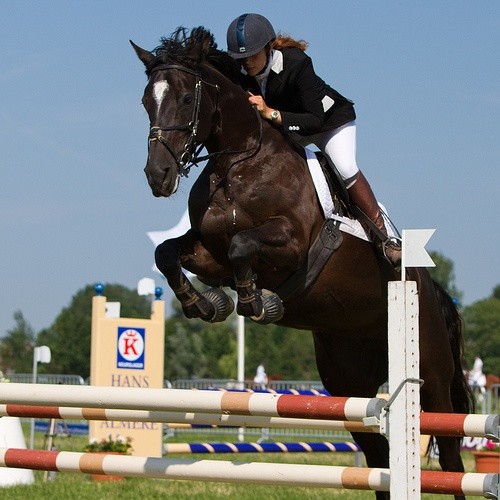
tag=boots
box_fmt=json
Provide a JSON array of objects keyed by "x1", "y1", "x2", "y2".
[{"x1": 346, "y1": 169, "x2": 401, "y2": 268}]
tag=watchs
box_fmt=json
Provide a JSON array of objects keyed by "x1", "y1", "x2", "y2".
[{"x1": 271, "y1": 110, "x2": 279, "y2": 122}]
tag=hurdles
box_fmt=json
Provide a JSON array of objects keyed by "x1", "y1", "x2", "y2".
[{"x1": 0, "y1": 278, "x2": 499, "y2": 500}]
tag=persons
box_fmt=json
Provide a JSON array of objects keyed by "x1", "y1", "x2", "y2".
[
  {"x1": 256, "y1": 363, "x2": 268, "y2": 386},
  {"x1": 227, "y1": 13, "x2": 402, "y2": 267},
  {"x1": 470, "y1": 353, "x2": 483, "y2": 384}
]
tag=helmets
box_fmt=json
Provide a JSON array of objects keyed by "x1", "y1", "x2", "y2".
[{"x1": 226, "y1": 13, "x2": 276, "y2": 59}]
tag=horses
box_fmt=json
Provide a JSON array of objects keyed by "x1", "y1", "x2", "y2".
[{"x1": 129, "y1": 24, "x2": 469, "y2": 500}]
